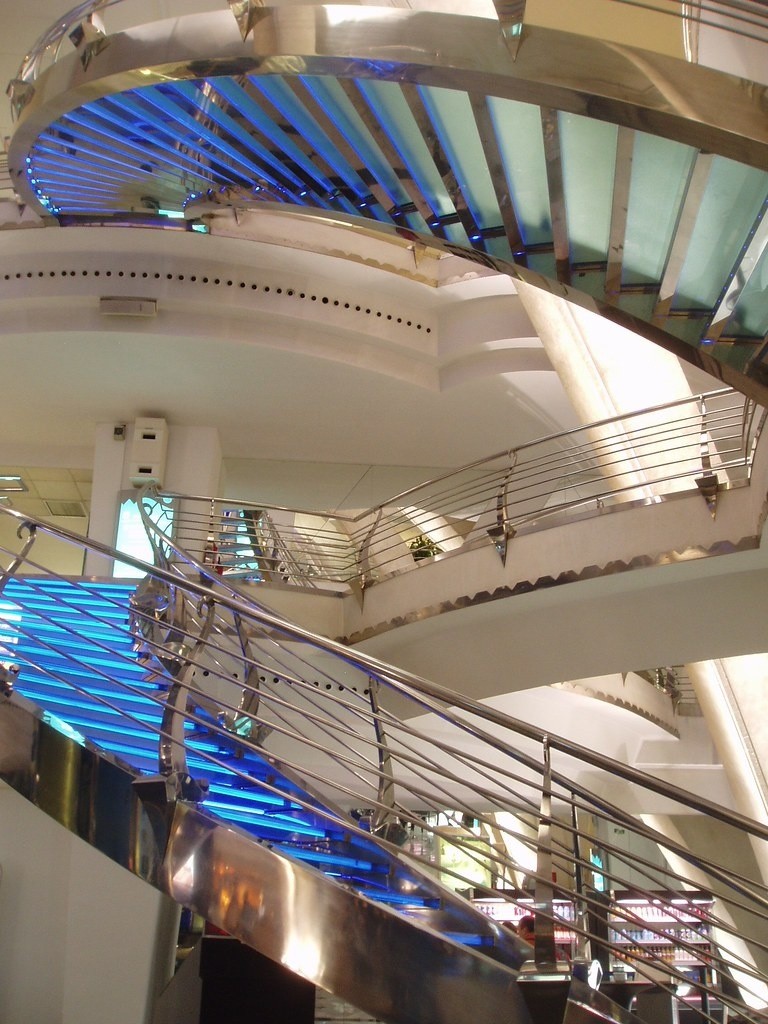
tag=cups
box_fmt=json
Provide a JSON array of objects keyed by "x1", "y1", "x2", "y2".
[{"x1": 613, "y1": 967, "x2": 625, "y2": 973}]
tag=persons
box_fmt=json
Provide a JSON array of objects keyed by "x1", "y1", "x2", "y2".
[{"x1": 517, "y1": 915, "x2": 573, "y2": 971}]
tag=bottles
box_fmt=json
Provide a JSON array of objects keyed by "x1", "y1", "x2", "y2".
[
  {"x1": 475, "y1": 903, "x2": 574, "y2": 918},
  {"x1": 609, "y1": 904, "x2": 709, "y2": 920},
  {"x1": 554, "y1": 922, "x2": 575, "y2": 943},
  {"x1": 611, "y1": 944, "x2": 710, "y2": 961},
  {"x1": 610, "y1": 923, "x2": 708, "y2": 941},
  {"x1": 683, "y1": 966, "x2": 699, "y2": 982}
]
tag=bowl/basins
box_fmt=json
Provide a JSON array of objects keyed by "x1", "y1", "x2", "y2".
[{"x1": 613, "y1": 972, "x2": 627, "y2": 982}]
